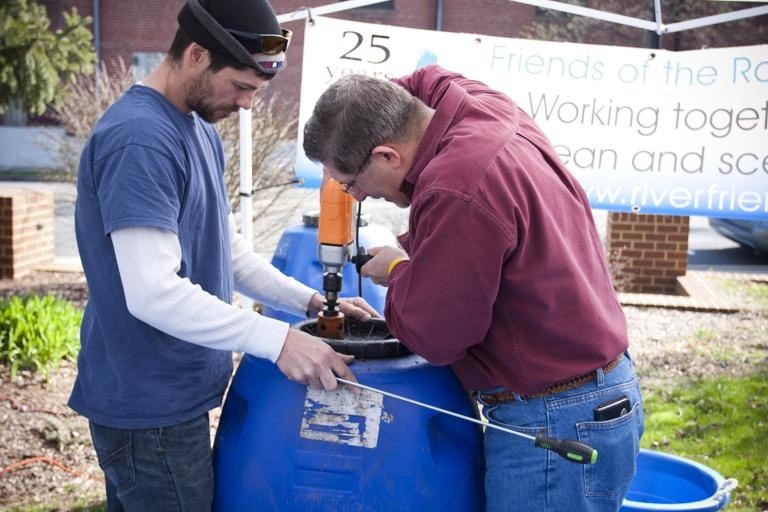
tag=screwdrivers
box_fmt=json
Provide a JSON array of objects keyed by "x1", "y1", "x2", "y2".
[{"x1": 332, "y1": 372, "x2": 600, "y2": 464}]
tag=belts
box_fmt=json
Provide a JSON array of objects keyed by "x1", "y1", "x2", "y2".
[{"x1": 471, "y1": 354, "x2": 626, "y2": 408}]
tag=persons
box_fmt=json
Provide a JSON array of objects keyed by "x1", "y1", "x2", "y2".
[
  {"x1": 65, "y1": 4, "x2": 387, "y2": 510},
  {"x1": 300, "y1": 61, "x2": 645, "y2": 512}
]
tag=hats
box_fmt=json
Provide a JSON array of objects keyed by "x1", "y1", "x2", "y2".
[{"x1": 176, "y1": 0, "x2": 289, "y2": 75}]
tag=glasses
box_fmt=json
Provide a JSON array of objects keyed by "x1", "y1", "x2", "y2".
[
  {"x1": 340, "y1": 145, "x2": 379, "y2": 195},
  {"x1": 227, "y1": 28, "x2": 293, "y2": 56}
]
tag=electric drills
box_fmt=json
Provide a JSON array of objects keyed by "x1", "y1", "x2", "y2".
[{"x1": 316, "y1": 176, "x2": 360, "y2": 337}]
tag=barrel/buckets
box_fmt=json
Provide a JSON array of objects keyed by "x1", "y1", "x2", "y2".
[
  {"x1": 620, "y1": 447, "x2": 739, "y2": 512},
  {"x1": 262, "y1": 211, "x2": 387, "y2": 325},
  {"x1": 213, "y1": 316, "x2": 485, "y2": 512}
]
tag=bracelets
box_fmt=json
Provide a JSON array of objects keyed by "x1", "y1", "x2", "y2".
[{"x1": 386, "y1": 256, "x2": 408, "y2": 273}]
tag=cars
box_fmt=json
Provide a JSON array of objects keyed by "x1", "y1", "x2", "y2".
[{"x1": 707, "y1": 216, "x2": 768, "y2": 264}]
